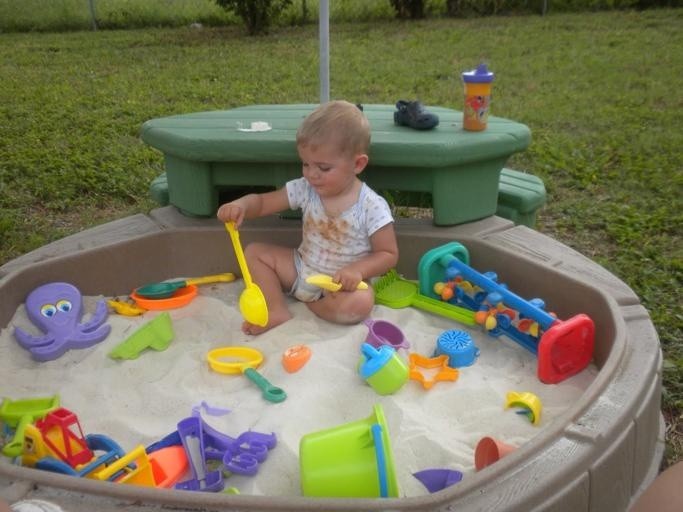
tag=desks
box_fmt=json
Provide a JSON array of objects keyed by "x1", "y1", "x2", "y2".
[{"x1": 137, "y1": 102, "x2": 532, "y2": 227}]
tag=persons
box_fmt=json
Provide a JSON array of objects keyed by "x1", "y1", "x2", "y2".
[{"x1": 216, "y1": 99, "x2": 400, "y2": 336}]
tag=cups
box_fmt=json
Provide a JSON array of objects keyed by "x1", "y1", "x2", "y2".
[{"x1": 461, "y1": 63, "x2": 495, "y2": 133}]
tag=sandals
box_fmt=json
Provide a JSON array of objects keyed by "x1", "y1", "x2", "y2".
[{"x1": 392, "y1": 101, "x2": 442, "y2": 131}]
